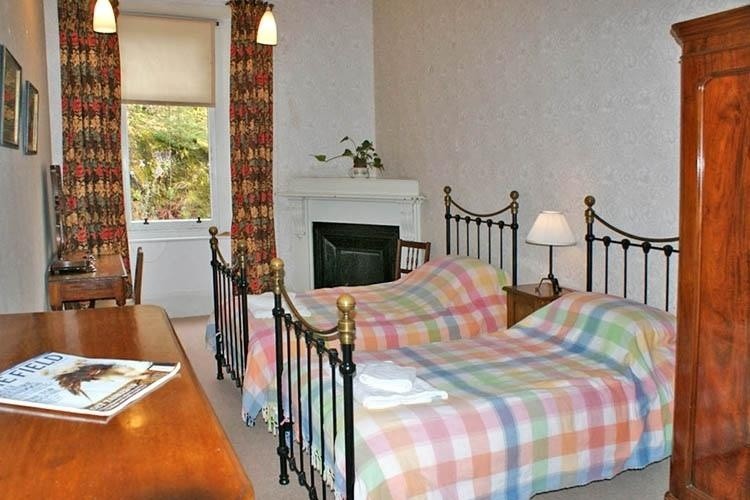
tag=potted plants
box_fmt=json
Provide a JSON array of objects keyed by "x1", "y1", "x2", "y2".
[{"x1": 308, "y1": 134, "x2": 387, "y2": 180}]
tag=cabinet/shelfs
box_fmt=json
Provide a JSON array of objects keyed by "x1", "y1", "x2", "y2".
[{"x1": 664, "y1": 1, "x2": 748, "y2": 499}]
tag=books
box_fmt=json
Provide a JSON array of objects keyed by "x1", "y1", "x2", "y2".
[
  {"x1": 0, "y1": 349, "x2": 183, "y2": 419},
  {"x1": 0, "y1": 369, "x2": 182, "y2": 426}
]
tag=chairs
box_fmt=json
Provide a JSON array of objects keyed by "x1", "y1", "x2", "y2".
[
  {"x1": 393, "y1": 237, "x2": 431, "y2": 284},
  {"x1": 125, "y1": 245, "x2": 146, "y2": 303}
]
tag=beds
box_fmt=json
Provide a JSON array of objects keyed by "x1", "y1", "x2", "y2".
[
  {"x1": 204, "y1": 187, "x2": 526, "y2": 426},
  {"x1": 266, "y1": 189, "x2": 691, "y2": 500}
]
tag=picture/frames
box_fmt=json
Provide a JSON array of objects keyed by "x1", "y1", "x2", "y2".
[
  {"x1": 25, "y1": 76, "x2": 41, "y2": 158},
  {"x1": 2, "y1": 44, "x2": 24, "y2": 153}
]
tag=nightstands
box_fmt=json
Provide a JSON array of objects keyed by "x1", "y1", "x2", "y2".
[{"x1": 501, "y1": 282, "x2": 573, "y2": 330}]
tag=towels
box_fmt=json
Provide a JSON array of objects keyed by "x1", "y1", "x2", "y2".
[
  {"x1": 236, "y1": 291, "x2": 312, "y2": 321},
  {"x1": 253, "y1": 287, "x2": 298, "y2": 310},
  {"x1": 321, "y1": 353, "x2": 450, "y2": 412},
  {"x1": 356, "y1": 361, "x2": 419, "y2": 394}
]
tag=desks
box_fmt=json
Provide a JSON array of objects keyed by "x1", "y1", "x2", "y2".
[
  {"x1": 46, "y1": 251, "x2": 131, "y2": 309},
  {"x1": 1, "y1": 304, "x2": 263, "y2": 499}
]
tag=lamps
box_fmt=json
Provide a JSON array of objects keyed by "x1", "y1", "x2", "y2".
[
  {"x1": 257, "y1": 1, "x2": 279, "y2": 48},
  {"x1": 90, "y1": 0, "x2": 121, "y2": 36},
  {"x1": 523, "y1": 207, "x2": 578, "y2": 296}
]
